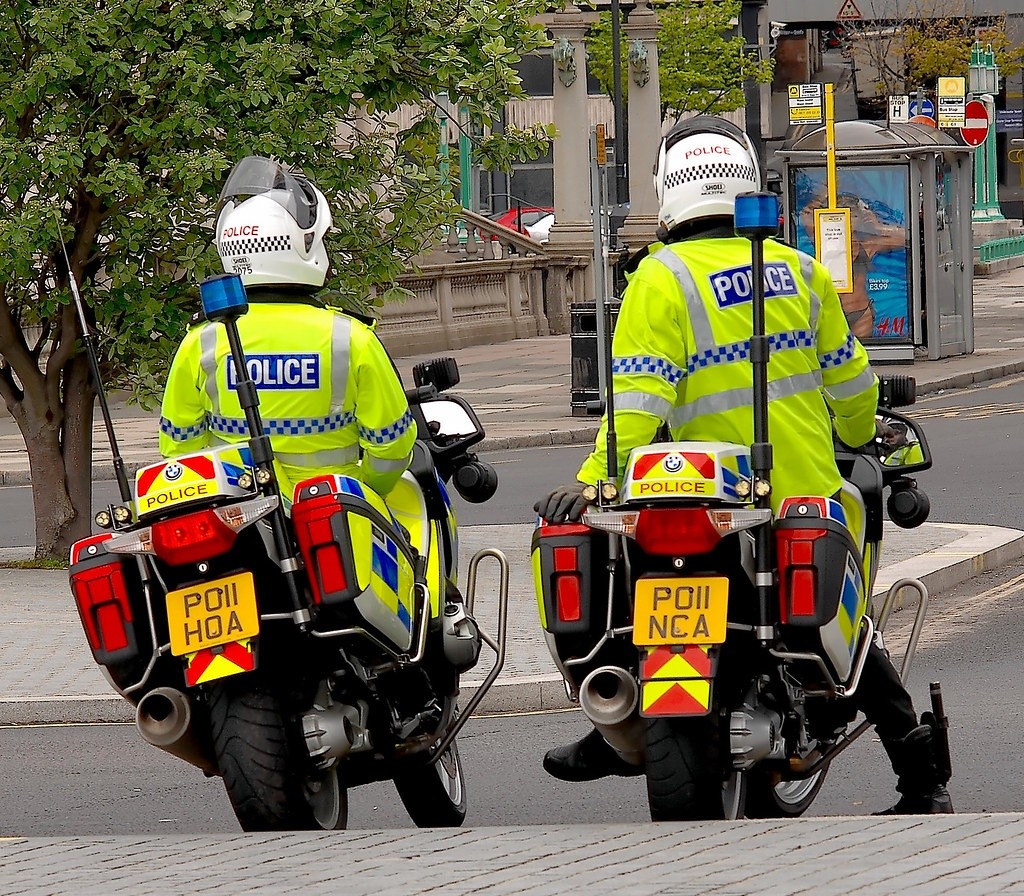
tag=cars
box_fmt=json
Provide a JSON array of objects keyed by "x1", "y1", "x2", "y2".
[{"x1": 480, "y1": 206, "x2": 555, "y2": 246}]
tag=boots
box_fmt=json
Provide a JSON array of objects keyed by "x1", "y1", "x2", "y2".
[
  {"x1": 543, "y1": 727, "x2": 644, "y2": 782},
  {"x1": 870, "y1": 711, "x2": 955, "y2": 816}
]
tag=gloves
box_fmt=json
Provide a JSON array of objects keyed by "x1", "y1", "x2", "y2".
[
  {"x1": 533, "y1": 482, "x2": 588, "y2": 524},
  {"x1": 874, "y1": 418, "x2": 895, "y2": 438}
]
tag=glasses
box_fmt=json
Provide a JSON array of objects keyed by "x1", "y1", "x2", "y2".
[{"x1": 895, "y1": 429, "x2": 903, "y2": 434}]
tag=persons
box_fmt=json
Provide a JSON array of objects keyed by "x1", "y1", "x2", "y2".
[
  {"x1": 801, "y1": 176, "x2": 905, "y2": 338},
  {"x1": 160, "y1": 158, "x2": 420, "y2": 496},
  {"x1": 882, "y1": 421, "x2": 924, "y2": 464},
  {"x1": 534, "y1": 117, "x2": 953, "y2": 815}
]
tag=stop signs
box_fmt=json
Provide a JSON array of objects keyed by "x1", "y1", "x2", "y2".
[{"x1": 960, "y1": 101, "x2": 989, "y2": 146}]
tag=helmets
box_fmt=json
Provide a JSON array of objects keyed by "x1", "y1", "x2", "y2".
[
  {"x1": 213, "y1": 156, "x2": 342, "y2": 291},
  {"x1": 652, "y1": 116, "x2": 763, "y2": 233},
  {"x1": 882, "y1": 416, "x2": 909, "y2": 429}
]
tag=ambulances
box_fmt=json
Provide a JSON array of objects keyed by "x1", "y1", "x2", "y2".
[
  {"x1": 67, "y1": 273, "x2": 508, "y2": 835},
  {"x1": 530, "y1": 187, "x2": 930, "y2": 822}
]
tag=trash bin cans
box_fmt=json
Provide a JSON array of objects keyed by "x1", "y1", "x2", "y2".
[{"x1": 569, "y1": 297, "x2": 622, "y2": 417}]
